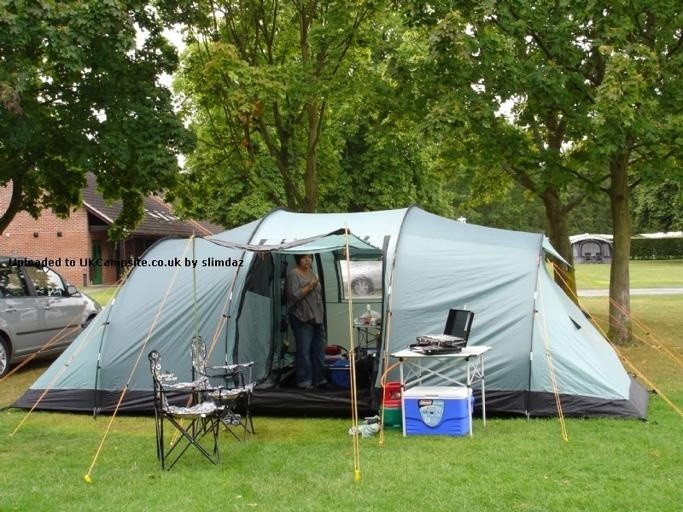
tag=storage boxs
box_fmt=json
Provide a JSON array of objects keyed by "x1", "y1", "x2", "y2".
[{"x1": 405, "y1": 386, "x2": 473, "y2": 437}]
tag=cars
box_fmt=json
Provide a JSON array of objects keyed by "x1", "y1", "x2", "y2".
[
  {"x1": 340, "y1": 259, "x2": 383, "y2": 296},
  {"x1": 0, "y1": 256, "x2": 103, "y2": 377}
]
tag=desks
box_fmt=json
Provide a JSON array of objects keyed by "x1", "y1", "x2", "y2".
[
  {"x1": 389, "y1": 346, "x2": 491, "y2": 437},
  {"x1": 352, "y1": 322, "x2": 381, "y2": 360}
]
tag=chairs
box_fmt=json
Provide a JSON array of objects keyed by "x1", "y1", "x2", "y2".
[
  {"x1": 148, "y1": 350, "x2": 224, "y2": 471},
  {"x1": 189, "y1": 336, "x2": 256, "y2": 442}
]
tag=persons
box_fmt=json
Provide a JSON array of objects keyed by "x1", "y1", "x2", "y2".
[{"x1": 286, "y1": 253, "x2": 337, "y2": 393}]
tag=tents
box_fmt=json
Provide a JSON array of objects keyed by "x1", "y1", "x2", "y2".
[{"x1": 1, "y1": 204, "x2": 659, "y2": 426}]
